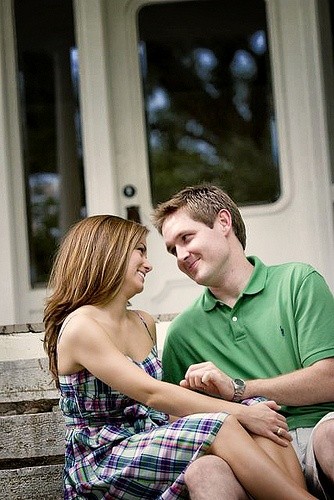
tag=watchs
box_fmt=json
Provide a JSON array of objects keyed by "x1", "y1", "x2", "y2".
[{"x1": 232, "y1": 378, "x2": 244, "y2": 403}]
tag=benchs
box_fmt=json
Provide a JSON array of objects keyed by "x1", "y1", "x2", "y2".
[{"x1": 0, "y1": 313, "x2": 182, "y2": 499}]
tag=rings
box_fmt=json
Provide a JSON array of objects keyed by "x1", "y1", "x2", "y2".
[{"x1": 276, "y1": 426, "x2": 283, "y2": 436}]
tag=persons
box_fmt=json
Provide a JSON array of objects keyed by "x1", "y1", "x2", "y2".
[
  {"x1": 42, "y1": 215, "x2": 334, "y2": 500},
  {"x1": 147, "y1": 183, "x2": 334, "y2": 499}
]
